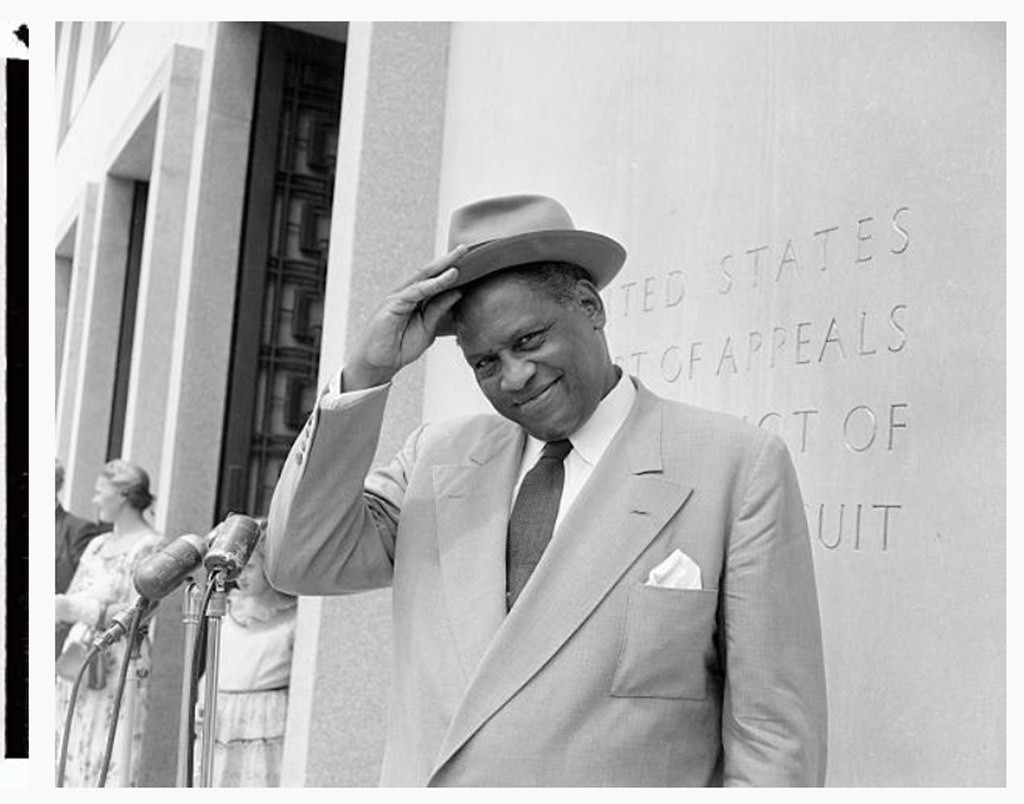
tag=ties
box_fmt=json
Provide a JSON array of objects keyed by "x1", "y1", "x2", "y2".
[{"x1": 505, "y1": 439, "x2": 572, "y2": 610}]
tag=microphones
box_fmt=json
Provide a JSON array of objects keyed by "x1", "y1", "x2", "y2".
[
  {"x1": 205, "y1": 514, "x2": 261, "y2": 620},
  {"x1": 92, "y1": 534, "x2": 207, "y2": 652}
]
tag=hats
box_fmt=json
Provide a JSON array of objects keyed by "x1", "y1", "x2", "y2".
[{"x1": 421, "y1": 194, "x2": 626, "y2": 336}]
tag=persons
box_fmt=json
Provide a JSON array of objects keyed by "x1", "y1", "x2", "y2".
[
  {"x1": 262, "y1": 195, "x2": 830, "y2": 787},
  {"x1": 191, "y1": 518, "x2": 298, "y2": 788},
  {"x1": 55, "y1": 458, "x2": 169, "y2": 788}
]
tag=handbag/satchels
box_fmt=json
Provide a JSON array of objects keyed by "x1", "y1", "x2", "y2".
[{"x1": 56, "y1": 599, "x2": 113, "y2": 689}]
